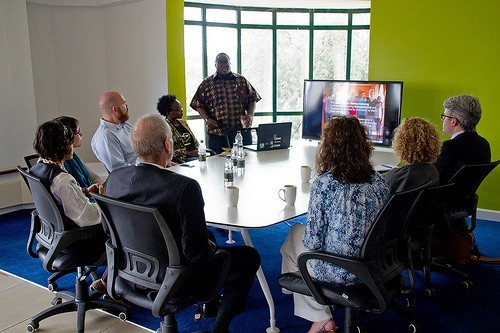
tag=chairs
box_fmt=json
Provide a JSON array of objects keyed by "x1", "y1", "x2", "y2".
[{"x1": 16, "y1": 154, "x2": 500, "y2": 333}]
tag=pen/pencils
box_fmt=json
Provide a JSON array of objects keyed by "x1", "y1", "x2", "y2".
[
  {"x1": 381, "y1": 164, "x2": 392, "y2": 168},
  {"x1": 180, "y1": 164, "x2": 196, "y2": 167}
]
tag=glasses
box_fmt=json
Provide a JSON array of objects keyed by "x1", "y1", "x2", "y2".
[
  {"x1": 170, "y1": 104, "x2": 183, "y2": 112},
  {"x1": 441, "y1": 114, "x2": 461, "y2": 124},
  {"x1": 74, "y1": 127, "x2": 81, "y2": 136}
]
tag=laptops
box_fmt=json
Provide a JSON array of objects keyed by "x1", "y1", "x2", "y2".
[{"x1": 243, "y1": 122, "x2": 292, "y2": 152}]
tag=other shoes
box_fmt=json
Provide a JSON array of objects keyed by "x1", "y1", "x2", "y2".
[{"x1": 92, "y1": 280, "x2": 106, "y2": 293}]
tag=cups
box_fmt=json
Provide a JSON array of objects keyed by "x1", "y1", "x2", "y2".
[
  {"x1": 227, "y1": 186, "x2": 239, "y2": 207},
  {"x1": 227, "y1": 207, "x2": 238, "y2": 224},
  {"x1": 301, "y1": 166, "x2": 316, "y2": 183},
  {"x1": 278, "y1": 185, "x2": 296, "y2": 206}
]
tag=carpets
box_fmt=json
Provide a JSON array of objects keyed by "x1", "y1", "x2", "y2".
[{"x1": 0, "y1": 204, "x2": 500, "y2": 333}]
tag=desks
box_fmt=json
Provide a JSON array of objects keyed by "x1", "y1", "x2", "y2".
[{"x1": 164, "y1": 139, "x2": 402, "y2": 333}]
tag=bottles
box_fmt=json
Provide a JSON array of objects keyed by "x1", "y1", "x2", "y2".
[
  {"x1": 235, "y1": 131, "x2": 243, "y2": 145},
  {"x1": 230, "y1": 143, "x2": 245, "y2": 176},
  {"x1": 223, "y1": 155, "x2": 233, "y2": 190},
  {"x1": 198, "y1": 140, "x2": 206, "y2": 163}
]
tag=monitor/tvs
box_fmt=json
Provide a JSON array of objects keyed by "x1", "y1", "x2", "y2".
[{"x1": 302, "y1": 79, "x2": 404, "y2": 147}]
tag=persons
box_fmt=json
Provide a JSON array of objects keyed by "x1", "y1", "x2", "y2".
[
  {"x1": 280, "y1": 115, "x2": 390, "y2": 333},
  {"x1": 433, "y1": 95, "x2": 492, "y2": 185},
  {"x1": 28, "y1": 121, "x2": 107, "y2": 294},
  {"x1": 91, "y1": 91, "x2": 176, "y2": 172},
  {"x1": 157, "y1": 94, "x2": 199, "y2": 162},
  {"x1": 381, "y1": 117, "x2": 439, "y2": 193},
  {"x1": 106, "y1": 112, "x2": 261, "y2": 333},
  {"x1": 54, "y1": 116, "x2": 104, "y2": 203},
  {"x1": 190, "y1": 52, "x2": 262, "y2": 155},
  {"x1": 347, "y1": 90, "x2": 384, "y2": 141}
]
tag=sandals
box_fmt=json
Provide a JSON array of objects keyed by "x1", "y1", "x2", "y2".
[{"x1": 316, "y1": 319, "x2": 339, "y2": 333}]
